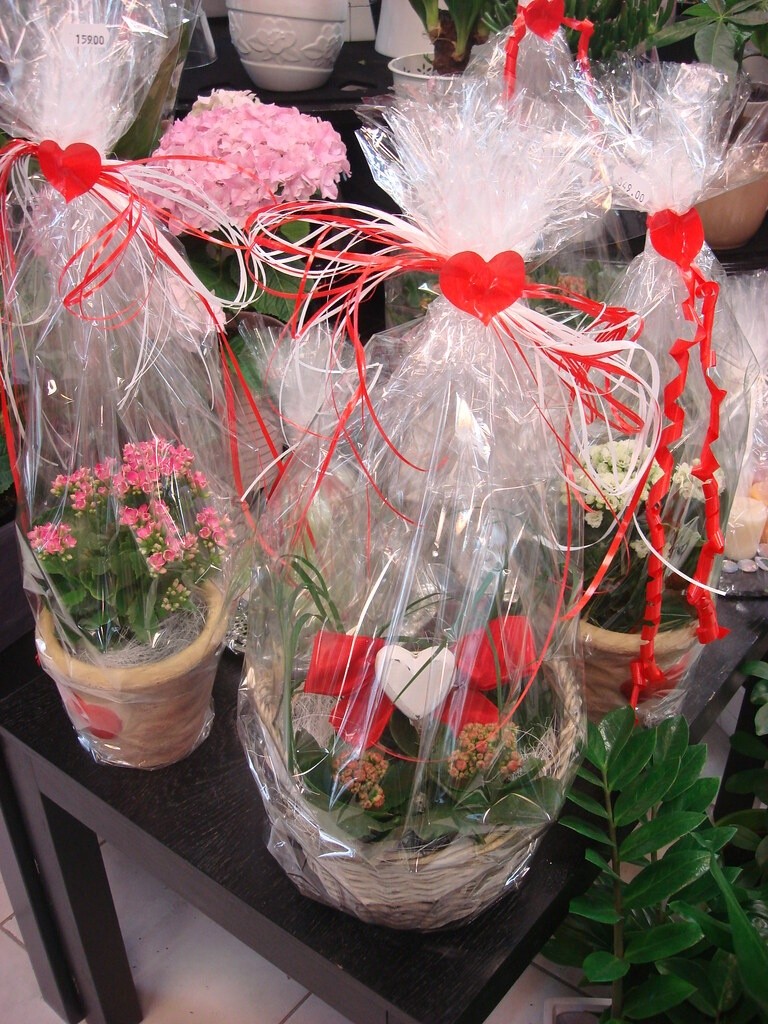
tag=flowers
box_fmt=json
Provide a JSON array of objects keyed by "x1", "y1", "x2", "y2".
[
  {"x1": 261, "y1": 555, "x2": 565, "y2": 849},
  {"x1": 544, "y1": 432, "x2": 735, "y2": 640},
  {"x1": 143, "y1": 88, "x2": 350, "y2": 391},
  {"x1": 21, "y1": 438, "x2": 234, "y2": 663}
]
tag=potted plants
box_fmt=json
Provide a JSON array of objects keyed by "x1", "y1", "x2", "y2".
[
  {"x1": 544, "y1": 707, "x2": 767, "y2": 1024},
  {"x1": 387, "y1": 0, "x2": 675, "y2": 143},
  {"x1": 645, "y1": 0, "x2": 768, "y2": 248}
]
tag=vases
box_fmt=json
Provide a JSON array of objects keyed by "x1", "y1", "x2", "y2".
[
  {"x1": 226, "y1": 0, "x2": 349, "y2": 96},
  {"x1": 32, "y1": 580, "x2": 232, "y2": 769},
  {"x1": 533, "y1": 600, "x2": 705, "y2": 732}
]
